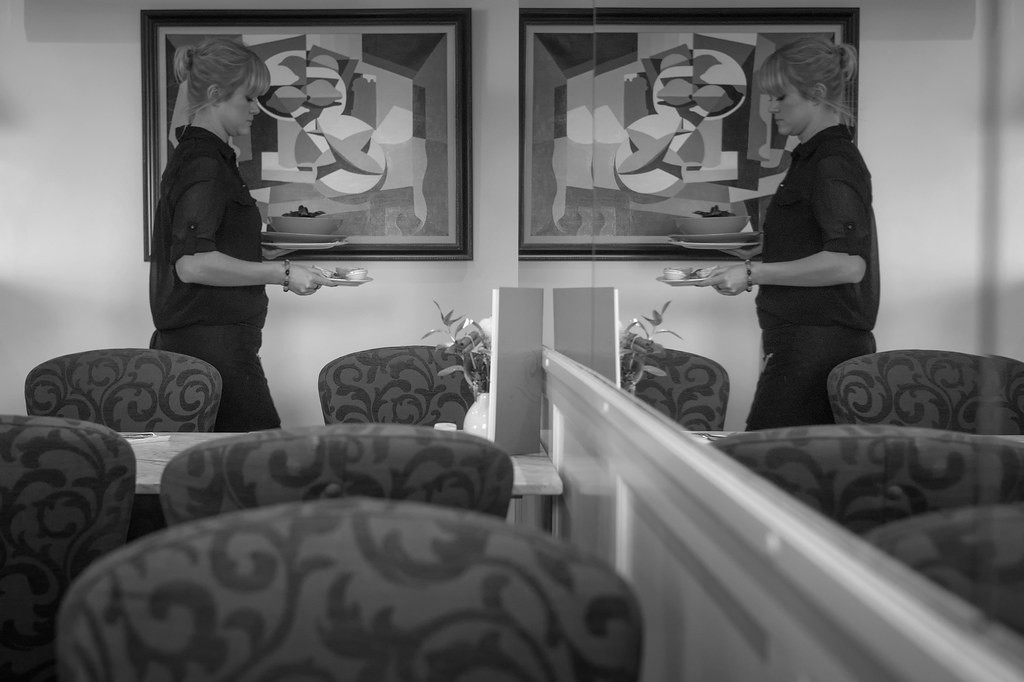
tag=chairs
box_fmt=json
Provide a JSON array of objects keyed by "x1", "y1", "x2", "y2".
[
  {"x1": 827, "y1": 349, "x2": 1024, "y2": 435},
  {"x1": 634, "y1": 346, "x2": 729, "y2": 430},
  {"x1": 52, "y1": 501, "x2": 645, "y2": 682},
  {"x1": 158, "y1": 423, "x2": 513, "y2": 530},
  {"x1": 862, "y1": 504, "x2": 1024, "y2": 640},
  {"x1": 709, "y1": 424, "x2": 1024, "y2": 539},
  {"x1": 318, "y1": 346, "x2": 490, "y2": 428},
  {"x1": 0, "y1": 414, "x2": 137, "y2": 682},
  {"x1": 25, "y1": 348, "x2": 222, "y2": 432}
]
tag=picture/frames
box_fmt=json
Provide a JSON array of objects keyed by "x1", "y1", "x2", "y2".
[
  {"x1": 140, "y1": 8, "x2": 474, "y2": 262},
  {"x1": 519, "y1": 8, "x2": 860, "y2": 261}
]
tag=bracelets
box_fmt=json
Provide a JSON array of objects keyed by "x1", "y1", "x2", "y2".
[
  {"x1": 283, "y1": 259, "x2": 290, "y2": 292},
  {"x1": 746, "y1": 259, "x2": 752, "y2": 292}
]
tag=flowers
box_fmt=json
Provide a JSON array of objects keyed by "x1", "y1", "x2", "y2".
[{"x1": 422, "y1": 298, "x2": 493, "y2": 402}]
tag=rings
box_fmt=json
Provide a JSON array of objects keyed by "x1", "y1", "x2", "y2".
[
  {"x1": 717, "y1": 286, "x2": 721, "y2": 290},
  {"x1": 314, "y1": 284, "x2": 318, "y2": 290}
]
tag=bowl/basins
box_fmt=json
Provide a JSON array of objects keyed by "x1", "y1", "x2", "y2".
[
  {"x1": 270, "y1": 216, "x2": 344, "y2": 233},
  {"x1": 672, "y1": 217, "x2": 751, "y2": 233}
]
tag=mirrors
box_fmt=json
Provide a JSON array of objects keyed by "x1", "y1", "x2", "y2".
[{"x1": 514, "y1": 0, "x2": 1024, "y2": 682}]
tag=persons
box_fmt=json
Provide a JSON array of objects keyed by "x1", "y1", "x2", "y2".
[
  {"x1": 149, "y1": 37, "x2": 338, "y2": 432},
  {"x1": 694, "y1": 36, "x2": 880, "y2": 431}
]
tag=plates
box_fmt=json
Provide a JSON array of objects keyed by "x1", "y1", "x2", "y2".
[
  {"x1": 657, "y1": 276, "x2": 708, "y2": 286},
  {"x1": 261, "y1": 231, "x2": 348, "y2": 241},
  {"x1": 262, "y1": 242, "x2": 346, "y2": 249},
  {"x1": 667, "y1": 240, "x2": 762, "y2": 248},
  {"x1": 326, "y1": 276, "x2": 374, "y2": 286},
  {"x1": 668, "y1": 232, "x2": 764, "y2": 241}
]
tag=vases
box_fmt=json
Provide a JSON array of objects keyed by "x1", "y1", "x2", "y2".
[{"x1": 463, "y1": 392, "x2": 489, "y2": 441}]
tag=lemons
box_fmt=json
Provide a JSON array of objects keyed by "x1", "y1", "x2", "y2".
[
  {"x1": 696, "y1": 265, "x2": 720, "y2": 277},
  {"x1": 312, "y1": 264, "x2": 332, "y2": 277}
]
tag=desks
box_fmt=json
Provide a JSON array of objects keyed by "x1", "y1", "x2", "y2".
[{"x1": 117, "y1": 431, "x2": 563, "y2": 528}]
